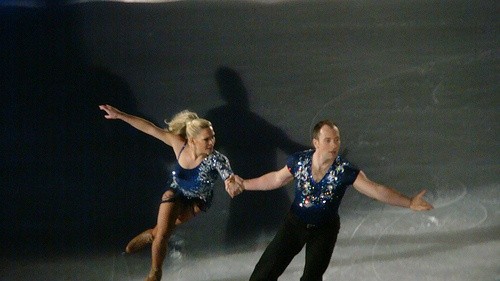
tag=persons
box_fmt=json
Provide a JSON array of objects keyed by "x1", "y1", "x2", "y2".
[
  {"x1": 224, "y1": 118, "x2": 436, "y2": 281},
  {"x1": 98, "y1": 103, "x2": 244, "y2": 281}
]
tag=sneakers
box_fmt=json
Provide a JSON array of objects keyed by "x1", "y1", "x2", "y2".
[
  {"x1": 125, "y1": 229, "x2": 154, "y2": 253},
  {"x1": 144, "y1": 268, "x2": 163, "y2": 281}
]
